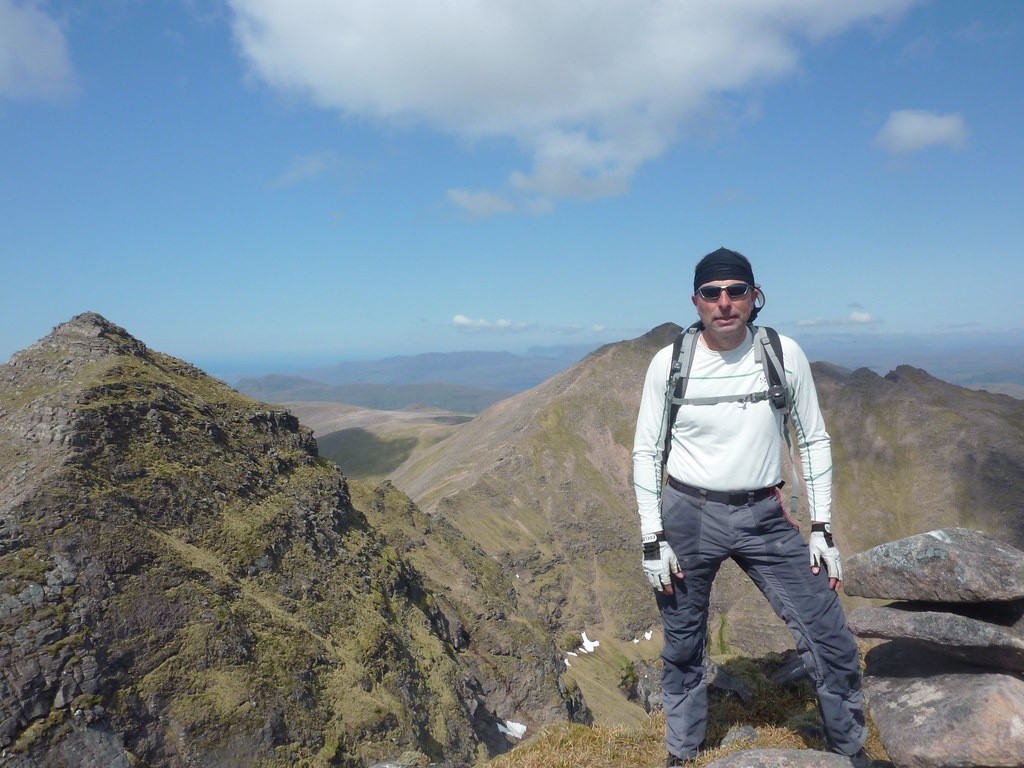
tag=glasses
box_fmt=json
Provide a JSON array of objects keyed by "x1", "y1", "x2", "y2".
[{"x1": 695, "y1": 283, "x2": 754, "y2": 299}]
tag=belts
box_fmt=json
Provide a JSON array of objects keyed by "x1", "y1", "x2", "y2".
[{"x1": 665, "y1": 475, "x2": 785, "y2": 507}]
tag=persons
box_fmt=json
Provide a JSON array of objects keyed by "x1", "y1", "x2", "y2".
[{"x1": 631, "y1": 248, "x2": 873, "y2": 768}]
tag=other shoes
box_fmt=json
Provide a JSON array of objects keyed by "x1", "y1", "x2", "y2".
[
  {"x1": 851, "y1": 747, "x2": 877, "y2": 768},
  {"x1": 665, "y1": 751, "x2": 695, "y2": 767}
]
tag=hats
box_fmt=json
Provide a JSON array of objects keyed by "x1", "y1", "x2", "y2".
[{"x1": 693, "y1": 248, "x2": 754, "y2": 292}]
